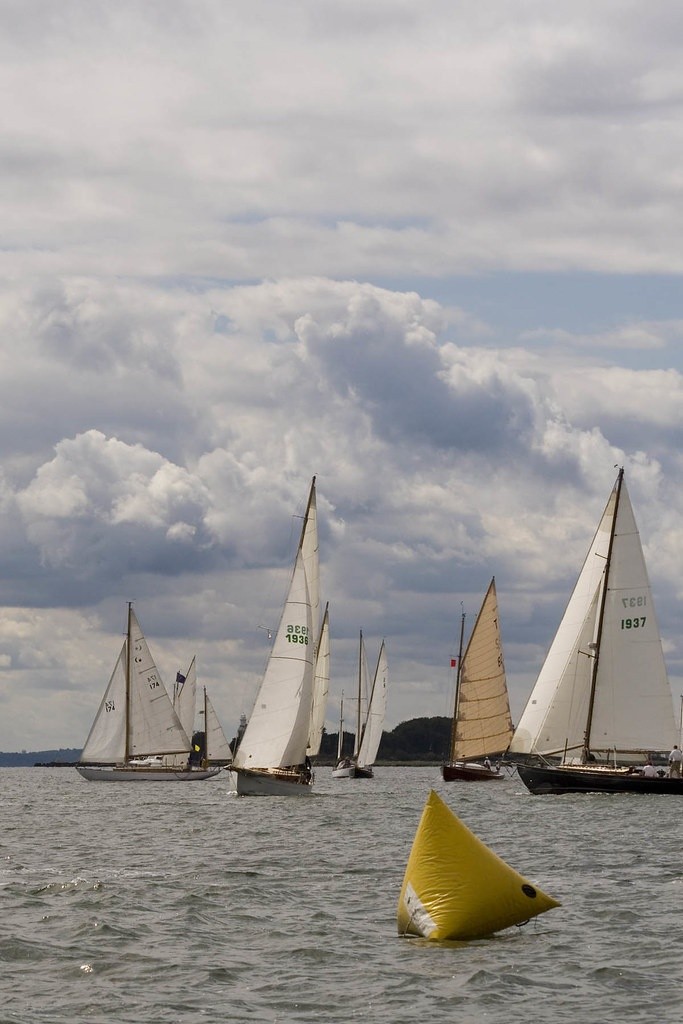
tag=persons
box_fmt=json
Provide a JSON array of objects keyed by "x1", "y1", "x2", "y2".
[
  {"x1": 668, "y1": 745, "x2": 681, "y2": 779},
  {"x1": 484, "y1": 762, "x2": 490, "y2": 769},
  {"x1": 495, "y1": 760, "x2": 500, "y2": 775},
  {"x1": 305, "y1": 766, "x2": 311, "y2": 781},
  {"x1": 642, "y1": 760, "x2": 658, "y2": 778}
]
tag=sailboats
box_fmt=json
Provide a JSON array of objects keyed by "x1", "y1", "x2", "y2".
[
  {"x1": 221, "y1": 475, "x2": 330, "y2": 798},
  {"x1": 438, "y1": 573, "x2": 515, "y2": 783},
  {"x1": 74, "y1": 600, "x2": 234, "y2": 781},
  {"x1": 511, "y1": 463, "x2": 682, "y2": 795},
  {"x1": 330, "y1": 628, "x2": 389, "y2": 779}
]
